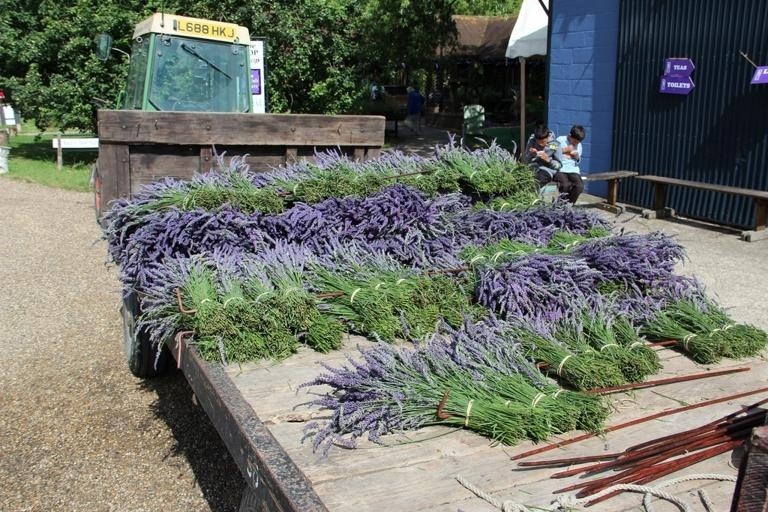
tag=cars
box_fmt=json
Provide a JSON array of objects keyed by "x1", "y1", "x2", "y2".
[{"x1": 374, "y1": 83, "x2": 410, "y2": 117}]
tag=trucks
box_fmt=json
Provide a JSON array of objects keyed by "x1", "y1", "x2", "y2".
[{"x1": 91, "y1": 13, "x2": 768, "y2": 512}]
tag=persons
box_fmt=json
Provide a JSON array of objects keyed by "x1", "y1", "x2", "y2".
[
  {"x1": 406, "y1": 86, "x2": 426, "y2": 137},
  {"x1": 524, "y1": 123, "x2": 563, "y2": 189},
  {"x1": 557, "y1": 123, "x2": 587, "y2": 205}
]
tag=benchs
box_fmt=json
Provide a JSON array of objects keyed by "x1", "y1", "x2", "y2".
[{"x1": 577, "y1": 165, "x2": 768, "y2": 243}]
